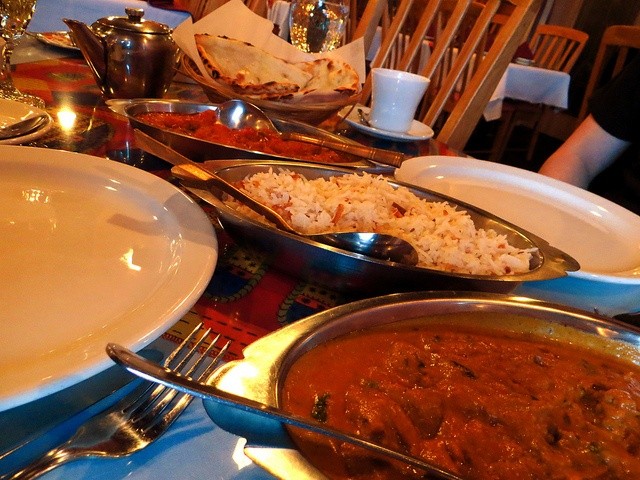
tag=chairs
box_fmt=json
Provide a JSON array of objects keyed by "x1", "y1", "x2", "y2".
[
  {"x1": 485, "y1": 12, "x2": 510, "y2": 35},
  {"x1": 381, "y1": 1, "x2": 444, "y2": 132},
  {"x1": 488, "y1": 23, "x2": 589, "y2": 167},
  {"x1": 348, "y1": 0, "x2": 543, "y2": 150},
  {"x1": 420, "y1": 0, "x2": 515, "y2": 163},
  {"x1": 524, "y1": 25, "x2": 639, "y2": 161},
  {"x1": 342, "y1": 0, "x2": 357, "y2": 46}
]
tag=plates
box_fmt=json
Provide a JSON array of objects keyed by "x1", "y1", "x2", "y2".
[
  {"x1": 171, "y1": 158, "x2": 580, "y2": 295},
  {"x1": 0, "y1": 143, "x2": 220, "y2": 414},
  {"x1": 0, "y1": 99, "x2": 54, "y2": 144},
  {"x1": 337, "y1": 105, "x2": 435, "y2": 143},
  {"x1": 36, "y1": 31, "x2": 81, "y2": 54}
]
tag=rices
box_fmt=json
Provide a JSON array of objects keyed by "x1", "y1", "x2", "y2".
[{"x1": 223, "y1": 168, "x2": 539, "y2": 277}]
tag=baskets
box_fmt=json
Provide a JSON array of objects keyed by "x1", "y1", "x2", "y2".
[{"x1": 181, "y1": 52, "x2": 363, "y2": 127}]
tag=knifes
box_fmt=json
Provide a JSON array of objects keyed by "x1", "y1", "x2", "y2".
[{"x1": 0, "y1": 348, "x2": 164, "y2": 460}]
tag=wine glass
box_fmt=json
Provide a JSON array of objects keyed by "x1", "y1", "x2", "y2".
[{"x1": 1, "y1": 0, "x2": 46, "y2": 108}]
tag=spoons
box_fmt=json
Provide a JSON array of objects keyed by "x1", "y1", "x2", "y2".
[
  {"x1": 0, "y1": 115, "x2": 47, "y2": 139},
  {"x1": 357, "y1": 108, "x2": 370, "y2": 127}
]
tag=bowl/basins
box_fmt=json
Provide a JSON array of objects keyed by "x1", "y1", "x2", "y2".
[
  {"x1": 202, "y1": 290, "x2": 640, "y2": 480},
  {"x1": 393, "y1": 155, "x2": 640, "y2": 318},
  {"x1": 106, "y1": 97, "x2": 395, "y2": 173}
]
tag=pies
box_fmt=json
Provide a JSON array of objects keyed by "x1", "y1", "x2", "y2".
[{"x1": 194, "y1": 32, "x2": 361, "y2": 100}]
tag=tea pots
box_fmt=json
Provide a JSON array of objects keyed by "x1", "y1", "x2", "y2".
[{"x1": 61, "y1": 7, "x2": 182, "y2": 100}]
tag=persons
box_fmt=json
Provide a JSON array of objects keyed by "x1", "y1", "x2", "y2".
[{"x1": 537, "y1": 45, "x2": 640, "y2": 216}]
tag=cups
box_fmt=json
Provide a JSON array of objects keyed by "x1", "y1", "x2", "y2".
[
  {"x1": 289, "y1": 0, "x2": 351, "y2": 54},
  {"x1": 368, "y1": 67, "x2": 430, "y2": 133}
]
